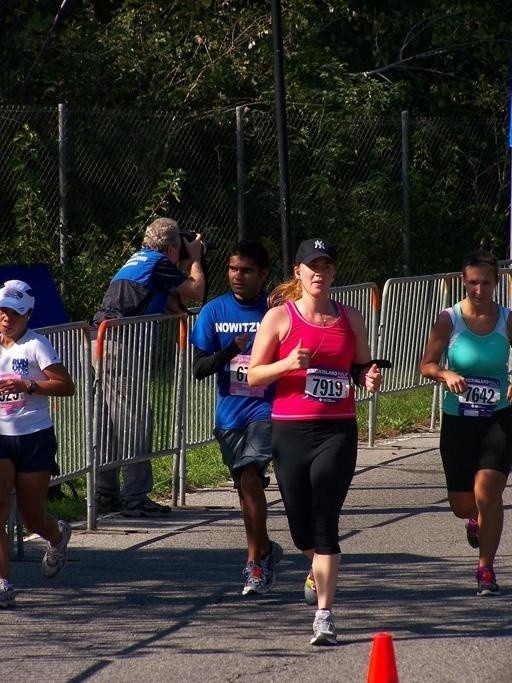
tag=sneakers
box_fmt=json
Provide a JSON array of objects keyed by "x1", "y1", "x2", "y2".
[
  {"x1": 304, "y1": 552, "x2": 340, "y2": 604},
  {"x1": 96, "y1": 492, "x2": 171, "y2": 518},
  {"x1": 0, "y1": 578, "x2": 18, "y2": 607},
  {"x1": 42, "y1": 520, "x2": 72, "y2": 577},
  {"x1": 467, "y1": 516, "x2": 480, "y2": 548},
  {"x1": 241, "y1": 541, "x2": 283, "y2": 595},
  {"x1": 310, "y1": 608, "x2": 337, "y2": 645},
  {"x1": 477, "y1": 566, "x2": 501, "y2": 596}
]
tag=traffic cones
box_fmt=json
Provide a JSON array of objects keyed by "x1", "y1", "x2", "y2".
[{"x1": 366, "y1": 633, "x2": 400, "y2": 683}]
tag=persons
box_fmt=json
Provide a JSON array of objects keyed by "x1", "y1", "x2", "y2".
[
  {"x1": 419, "y1": 252, "x2": 512, "y2": 597},
  {"x1": 248, "y1": 236, "x2": 382, "y2": 646},
  {"x1": 91, "y1": 216, "x2": 207, "y2": 520},
  {"x1": 0, "y1": 278, "x2": 72, "y2": 610},
  {"x1": 190, "y1": 241, "x2": 282, "y2": 599}
]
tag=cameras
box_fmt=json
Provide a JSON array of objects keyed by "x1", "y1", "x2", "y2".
[{"x1": 180, "y1": 232, "x2": 218, "y2": 259}]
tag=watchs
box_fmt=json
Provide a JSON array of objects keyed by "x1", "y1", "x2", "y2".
[{"x1": 27, "y1": 378, "x2": 38, "y2": 393}]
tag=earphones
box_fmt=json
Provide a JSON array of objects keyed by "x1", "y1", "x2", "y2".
[
  {"x1": 26, "y1": 316, "x2": 30, "y2": 321},
  {"x1": 296, "y1": 271, "x2": 300, "y2": 275}
]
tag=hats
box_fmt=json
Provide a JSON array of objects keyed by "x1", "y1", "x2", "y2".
[
  {"x1": 0, "y1": 279, "x2": 35, "y2": 315},
  {"x1": 295, "y1": 237, "x2": 337, "y2": 265}
]
